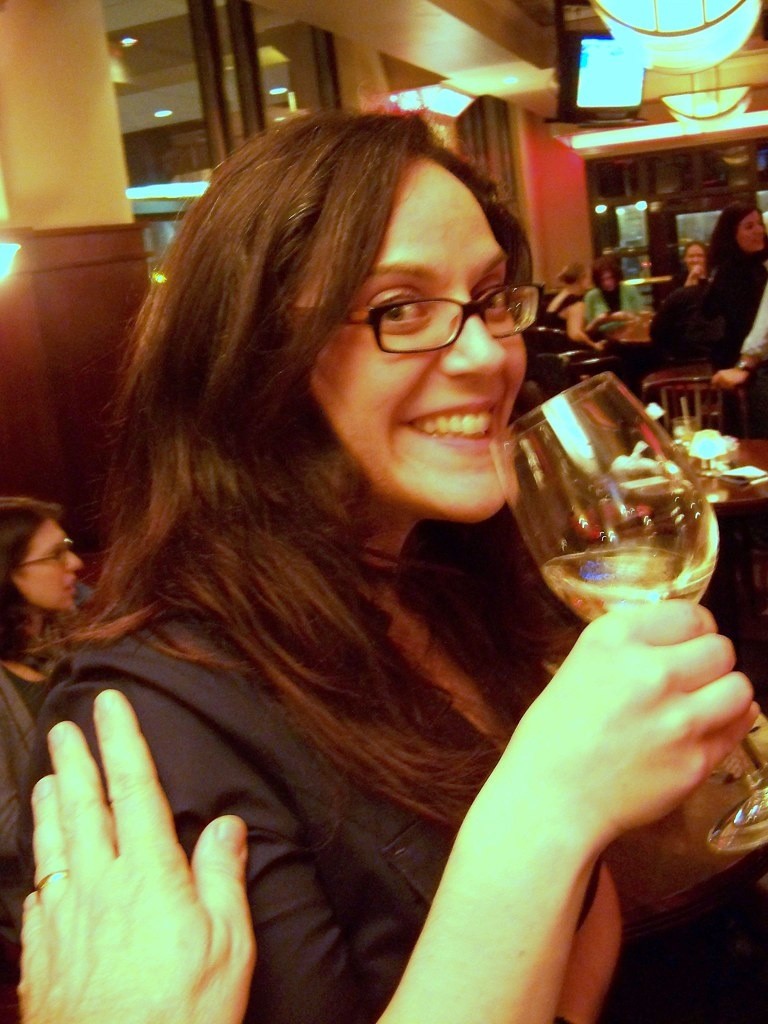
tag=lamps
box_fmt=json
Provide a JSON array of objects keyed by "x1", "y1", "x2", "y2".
[{"x1": 591, "y1": 0, "x2": 762, "y2": 131}]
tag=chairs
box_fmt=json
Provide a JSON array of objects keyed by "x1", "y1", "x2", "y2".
[{"x1": 641, "y1": 367, "x2": 750, "y2": 439}]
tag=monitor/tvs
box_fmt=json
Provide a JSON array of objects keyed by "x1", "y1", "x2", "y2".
[{"x1": 557, "y1": 30, "x2": 644, "y2": 120}]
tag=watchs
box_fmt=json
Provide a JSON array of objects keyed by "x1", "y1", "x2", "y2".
[{"x1": 737, "y1": 360, "x2": 752, "y2": 373}]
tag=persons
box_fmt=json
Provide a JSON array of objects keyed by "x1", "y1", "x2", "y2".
[
  {"x1": 32, "y1": 112, "x2": 767, "y2": 1023},
  {"x1": 17, "y1": 690, "x2": 256, "y2": 1024},
  {"x1": 1, "y1": 494, "x2": 85, "y2": 1024},
  {"x1": 539, "y1": 196, "x2": 768, "y2": 440}
]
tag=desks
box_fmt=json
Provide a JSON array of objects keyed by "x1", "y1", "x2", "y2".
[
  {"x1": 604, "y1": 312, "x2": 656, "y2": 386},
  {"x1": 675, "y1": 438, "x2": 768, "y2": 650}
]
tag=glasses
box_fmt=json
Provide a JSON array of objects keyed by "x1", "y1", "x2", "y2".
[
  {"x1": 342, "y1": 282, "x2": 545, "y2": 354},
  {"x1": 15, "y1": 539, "x2": 73, "y2": 566}
]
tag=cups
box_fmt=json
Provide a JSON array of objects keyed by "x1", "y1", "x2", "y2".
[{"x1": 662, "y1": 387, "x2": 699, "y2": 449}]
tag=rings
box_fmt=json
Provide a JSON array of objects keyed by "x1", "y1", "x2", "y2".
[{"x1": 34, "y1": 871, "x2": 70, "y2": 889}]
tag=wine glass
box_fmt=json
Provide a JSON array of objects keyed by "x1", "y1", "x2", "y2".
[{"x1": 491, "y1": 372, "x2": 768, "y2": 850}]
